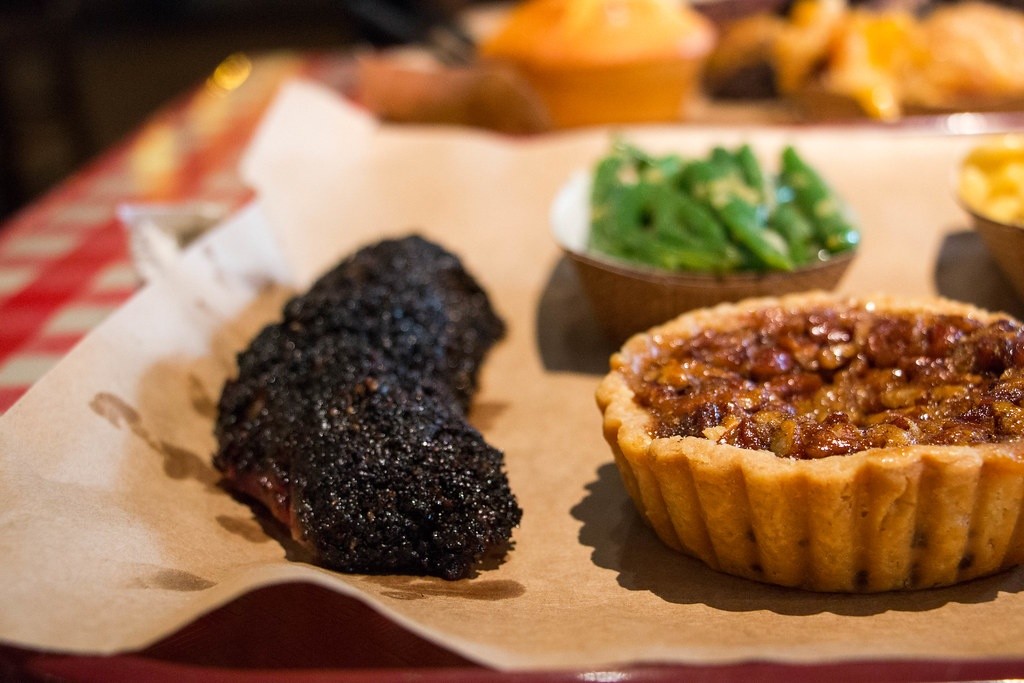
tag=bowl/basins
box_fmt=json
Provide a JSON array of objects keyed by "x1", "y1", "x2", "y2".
[
  {"x1": 552, "y1": 158, "x2": 864, "y2": 343},
  {"x1": 950, "y1": 137, "x2": 1024, "y2": 297}
]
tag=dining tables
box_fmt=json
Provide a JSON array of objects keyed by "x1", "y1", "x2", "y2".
[{"x1": 0, "y1": 50, "x2": 1023, "y2": 683}]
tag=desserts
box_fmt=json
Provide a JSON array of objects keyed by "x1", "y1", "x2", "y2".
[{"x1": 595, "y1": 286, "x2": 1024, "y2": 593}]
